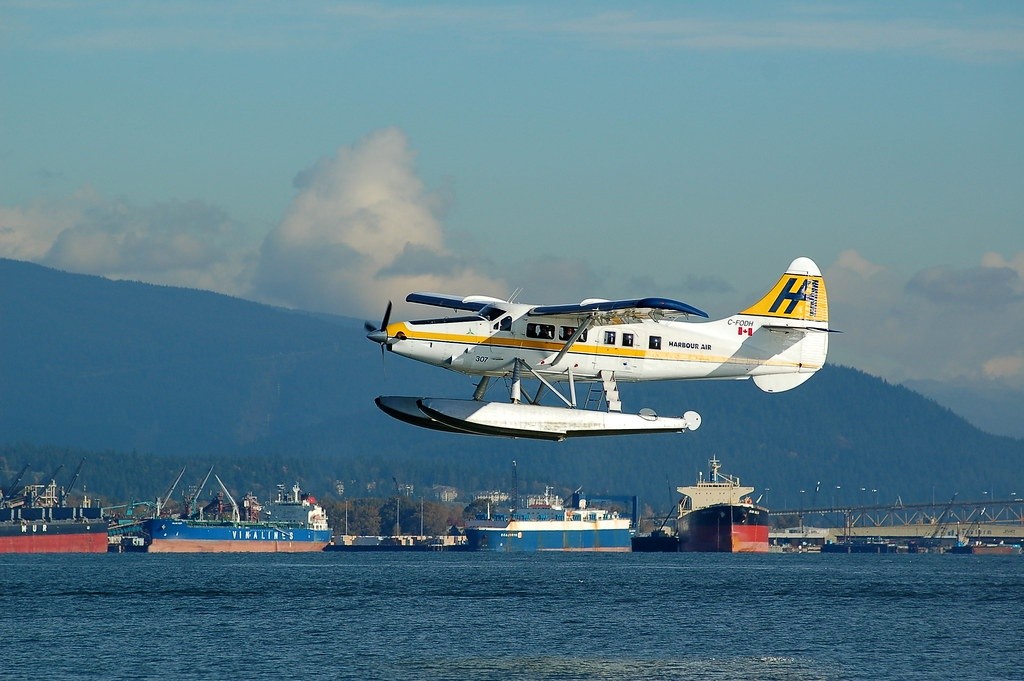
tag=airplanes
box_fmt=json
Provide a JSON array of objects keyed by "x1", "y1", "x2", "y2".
[{"x1": 364, "y1": 255, "x2": 846, "y2": 442}]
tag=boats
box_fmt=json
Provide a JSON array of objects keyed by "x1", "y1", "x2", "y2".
[
  {"x1": 631, "y1": 505, "x2": 679, "y2": 552},
  {"x1": 675, "y1": 452, "x2": 769, "y2": 553},
  {"x1": 464, "y1": 459, "x2": 632, "y2": 552},
  {"x1": 0, "y1": 456, "x2": 110, "y2": 554},
  {"x1": 142, "y1": 465, "x2": 333, "y2": 554}
]
